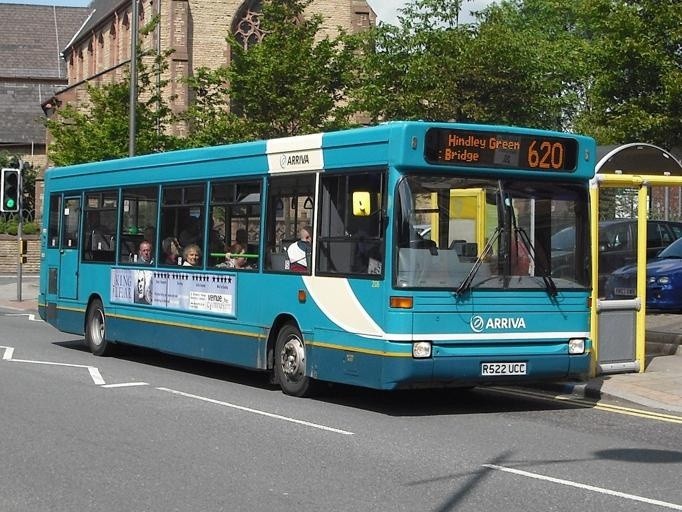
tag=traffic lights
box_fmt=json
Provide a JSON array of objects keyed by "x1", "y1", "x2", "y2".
[{"x1": 0, "y1": 169, "x2": 20, "y2": 213}]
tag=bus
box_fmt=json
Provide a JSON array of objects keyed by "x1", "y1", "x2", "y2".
[{"x1": 37, "y1": 120, "x2": 597, "y2": 398}]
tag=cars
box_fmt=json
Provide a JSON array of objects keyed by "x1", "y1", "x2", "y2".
[{"x1": 550, "y1": 218, "x2": 682, "y2": 314}]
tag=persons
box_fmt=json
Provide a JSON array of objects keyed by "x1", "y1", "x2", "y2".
[
  {"x1": 137, "y1": 226, "x2": 156, "y2": 266},
  {"x1": 137, "y1": 270, "x2": 149, "y2": 304},
  {"x1": 209, "y1": 217, "x2": 254, "y2": 270},
  {"x1": 358, "y1": 194, "x2": 423, "y2": 269},
  {"x1": 286, "y1": 227, "x2": 312, "y2": 272},
  {"x1": 182, "y1": 244, "x2": 202, "y2": 269}
]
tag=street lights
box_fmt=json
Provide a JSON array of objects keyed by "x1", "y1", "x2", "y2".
[{"x1": 7, "y1": 153, "x2": 33, "y2": 301}]
